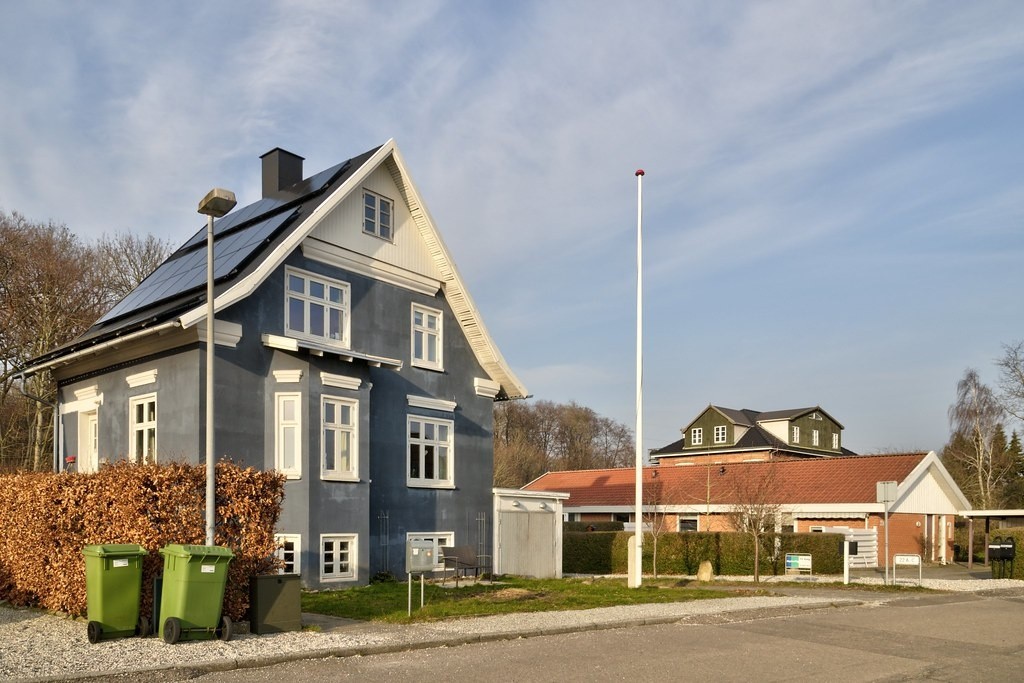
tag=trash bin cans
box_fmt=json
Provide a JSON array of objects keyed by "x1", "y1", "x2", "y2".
[
  {"x1": 82, "y1": 544, "x2": 148, "y2": 644},
  {"x1": 158, "y1": 544, "x2": 234, "y2": 644}
]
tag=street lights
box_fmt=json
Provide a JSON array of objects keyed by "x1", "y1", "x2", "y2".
[{"x1": 197, "y1": 187, "x2": 238, "y2": 546}]
